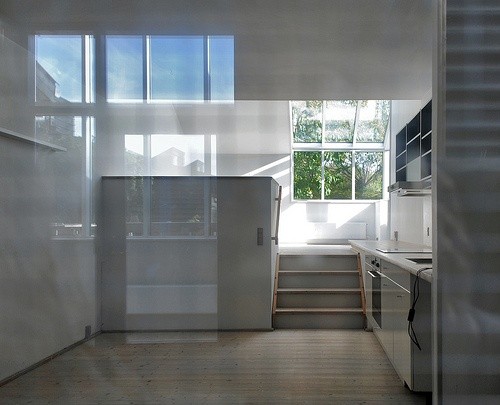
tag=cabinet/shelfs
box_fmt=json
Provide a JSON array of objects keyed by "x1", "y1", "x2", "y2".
[
  {"x1": 351, "y1": 236, "x2": 437, "y2": 393},
  {"x1": 391, "y1": 95, "x2": 435, "y2": 196}
]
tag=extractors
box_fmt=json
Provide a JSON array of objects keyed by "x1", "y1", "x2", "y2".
[{"x1": 387, "y1": 181, "x2": 432, "y2": 198}]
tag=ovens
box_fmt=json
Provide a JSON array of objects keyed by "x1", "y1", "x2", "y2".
[{"x1": 367, "y1": 256, "x2": 382, "y2": 328}]
